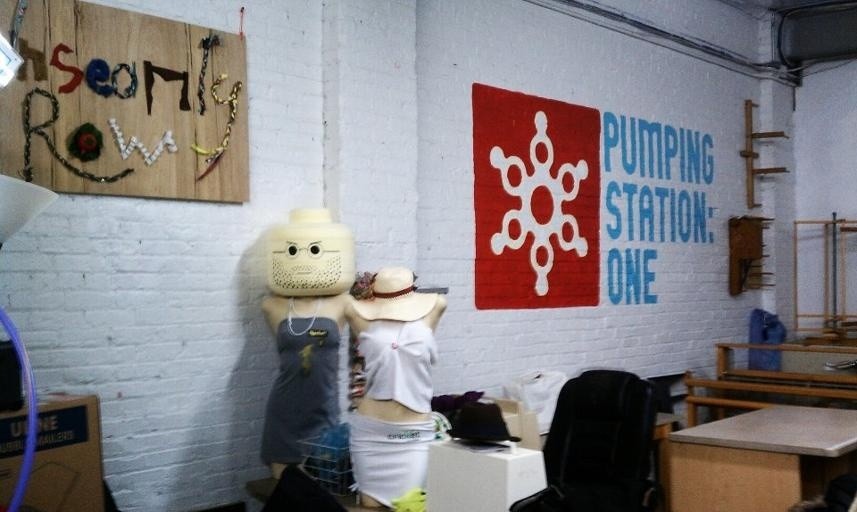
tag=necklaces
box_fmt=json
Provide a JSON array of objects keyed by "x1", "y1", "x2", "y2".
[{"x1": 287, "y1": 297, "x2": 322, "y2": 336}]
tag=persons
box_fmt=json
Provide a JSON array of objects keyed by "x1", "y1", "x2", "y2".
[
  {"x1": 343, "y1": 264, "x2": 449, "y2": 511},
  {"x1": 257, "y1": 205, "x2": 354, "y2": 483}
]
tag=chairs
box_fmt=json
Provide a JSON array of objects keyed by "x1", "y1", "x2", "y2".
[{"x1": 507, "y1": 368, "x2": 660, "y2": 511}]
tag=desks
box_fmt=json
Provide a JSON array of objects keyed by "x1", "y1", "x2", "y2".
[
  {"x1": 664, "y1": 398, "x2": 856, "y2": 512},
  {"x1": 657, "y1": 411, "x2": 688, "y2": 489}
]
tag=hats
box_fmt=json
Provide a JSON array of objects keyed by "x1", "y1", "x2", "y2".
[
  {"x1": 445, "y1": 401, "x2": 522, "y2": 442},
  {"x1": 351, "y1": 267, "x2": 438, "y2": 323}
]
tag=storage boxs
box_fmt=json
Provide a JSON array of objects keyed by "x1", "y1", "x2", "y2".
[{"x1": 1, "y1": 384, "x2": 106, "y2": 512}]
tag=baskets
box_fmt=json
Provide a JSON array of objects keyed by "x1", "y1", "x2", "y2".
[{"x1": 297, "y1": 435, "x2": 357, "y2": 497}]
